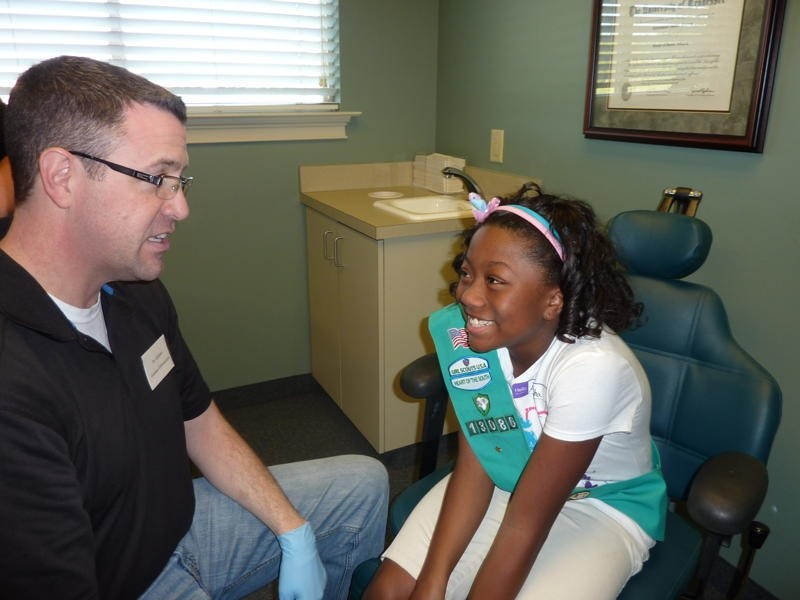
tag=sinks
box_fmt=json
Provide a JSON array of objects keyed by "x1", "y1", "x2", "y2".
[{"x1": 373, "y1": 195, "x2": 484, "y2": 221}]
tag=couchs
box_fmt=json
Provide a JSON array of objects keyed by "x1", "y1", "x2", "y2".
[{"x1": 387, "y1": 209, "x2": 784, "y2": 600}]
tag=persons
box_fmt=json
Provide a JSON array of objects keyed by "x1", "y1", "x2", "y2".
[
  {"x1": 359, "y1": 190, "x2": 670, "y2": 599},
  {"x1": 0, "y1": 54, "x2": 390, "y2": 600},
  {"x1": 0, "y1": 98, "x2": 19, "y2": 240}
]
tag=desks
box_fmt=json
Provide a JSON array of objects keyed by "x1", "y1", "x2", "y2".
[{"x1": 299, "y1": 160, "x2": 543, "y2": 453}]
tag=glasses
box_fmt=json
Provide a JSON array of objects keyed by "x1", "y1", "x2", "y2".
[{"x1": 68, "y1": 150, "x2": 195, "y2": 201}]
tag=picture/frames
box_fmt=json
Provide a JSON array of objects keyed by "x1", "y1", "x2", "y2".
[{"x1": 581, "y1": 1, "x2": 788, "y2": 153}]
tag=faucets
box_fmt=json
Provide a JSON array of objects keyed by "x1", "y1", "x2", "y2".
[{"x1": 440, "y1": 166, "x2": 486, "y2": 204}]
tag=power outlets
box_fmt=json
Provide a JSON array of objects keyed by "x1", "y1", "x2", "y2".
[{"x1": 489, "y1": 129, "x2": 504, "y2": 163}]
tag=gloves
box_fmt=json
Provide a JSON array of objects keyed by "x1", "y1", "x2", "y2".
[{"x1": 275, "y1": 521, "x2": 328, "y2": 600}]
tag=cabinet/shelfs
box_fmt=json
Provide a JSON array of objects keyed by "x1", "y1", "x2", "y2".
[{"x1": 304, "y1": 205, "x2": 386, "y2": 454}]
